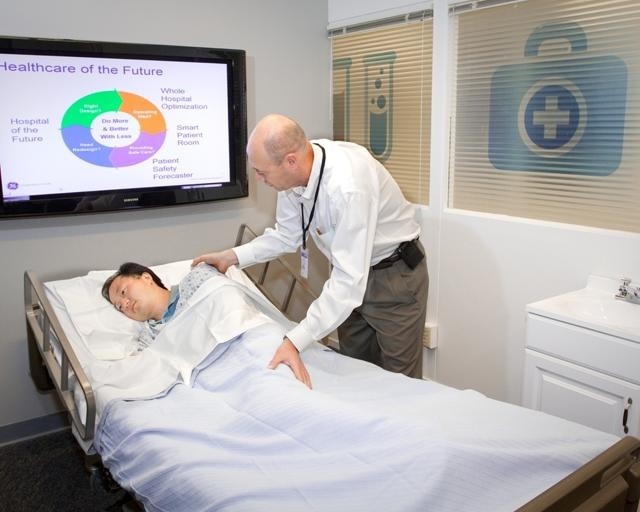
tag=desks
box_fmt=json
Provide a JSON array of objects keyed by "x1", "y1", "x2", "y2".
[{"x1": 521, "y1": 279, "x2": 640, "y2": 439}]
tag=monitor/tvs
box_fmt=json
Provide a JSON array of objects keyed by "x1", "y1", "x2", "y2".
[{"x1": 0, "y1": 34, "x2": 248, "y2": 221}]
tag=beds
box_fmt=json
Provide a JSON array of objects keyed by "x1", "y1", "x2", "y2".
[{"x1": 21, "y1": 219, "x2": 640, "y2": 511}]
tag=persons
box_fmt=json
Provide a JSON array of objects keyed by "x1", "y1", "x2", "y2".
[
  {"x1": 192, "y1": 115, "x2": 430, "y2": 389},
  {"x1": 101, "y1": 263, "x2": 224, "y2": 356}
]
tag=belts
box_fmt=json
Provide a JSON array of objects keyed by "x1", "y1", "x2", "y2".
[{"x1": 374, "y1": 255, "x2": 401, "y2": 269}]
